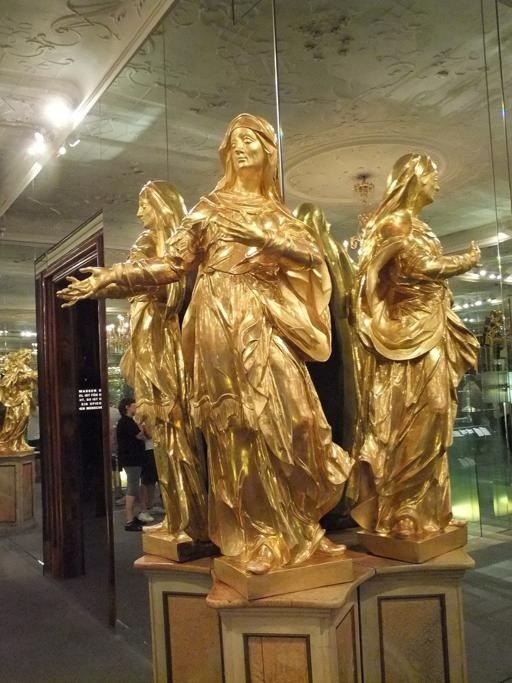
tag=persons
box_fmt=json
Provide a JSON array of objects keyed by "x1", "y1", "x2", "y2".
[
  {"x1": 68, "y1": 112, "x2": 356, "y2": 575},
  {"x1": 109, "y1": 398, "x2": 165, "y2": 531},
  {"x1": 484, "y1": 309, "x2": 512, "y2": 369},
  {"x1": 290, "y1": 201, "x2": 357, "y2": 446},
  {"x1": 52, "y1": 181, "x2": 189, "y2": 533},
  {"x1": 340, "y1": 151, "x2": 484, "y2": 538},
  {"x1": 0, "y1": 349, "x2": 38, "y2": 452}
]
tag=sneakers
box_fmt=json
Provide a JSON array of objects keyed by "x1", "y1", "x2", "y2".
[{"x1": 124, "y1": 505, "x2": 165, "y2": 533}]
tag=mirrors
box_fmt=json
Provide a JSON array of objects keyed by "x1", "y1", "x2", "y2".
[{"x1": 0, "y1": 2, "x2": 510, "y2": 682}]
